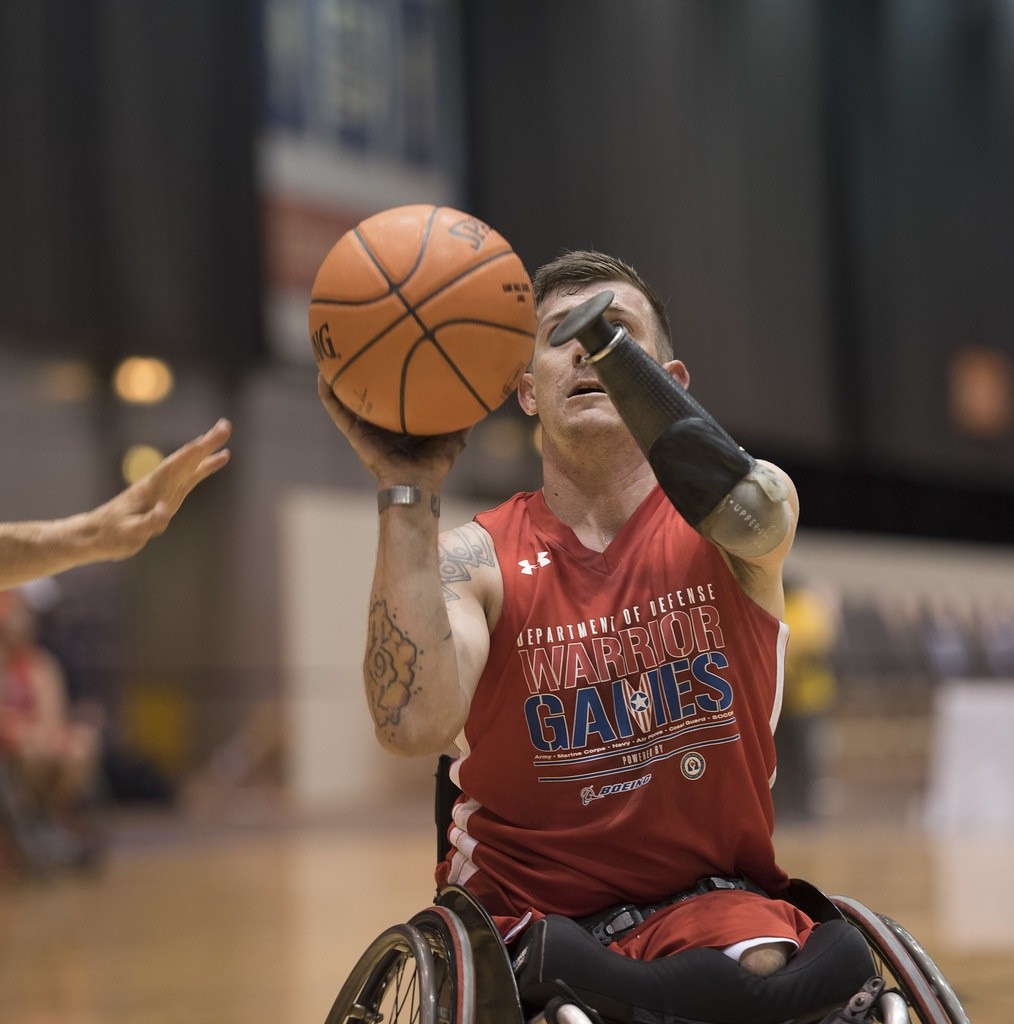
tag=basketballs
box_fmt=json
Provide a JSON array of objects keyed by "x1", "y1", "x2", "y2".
[{"x1": 308, "y1": 203, "x2": 539, "y2": 435}]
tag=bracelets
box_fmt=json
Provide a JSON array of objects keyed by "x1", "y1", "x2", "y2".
[
  {"x1": 582, "y1": 326, "x2": 626, "y2": 365},
  {"x1": 377, "y1": 483, "x2": 443, "y2": 518}
]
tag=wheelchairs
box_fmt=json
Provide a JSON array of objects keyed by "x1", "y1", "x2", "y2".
[{"x1": 319, "y1": 749, "x2": 971, "y2": 1024}]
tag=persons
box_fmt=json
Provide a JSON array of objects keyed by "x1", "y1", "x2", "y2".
[
  {"x1": 317, "y1": 251, "x2": 888, "y2": 1024},
  {"x1": 0, "y1": 417, "x2": 230, "y2": 592}
]
